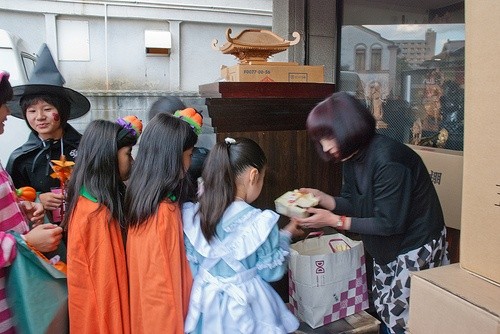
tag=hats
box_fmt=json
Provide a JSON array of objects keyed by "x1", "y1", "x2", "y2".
[{"x1": 6, "y1": 44, "x2": 91, "y2": 120}]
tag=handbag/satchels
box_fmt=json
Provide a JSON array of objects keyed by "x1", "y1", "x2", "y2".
[
  {"x1": 288, "y1": 231, "x2": 370, "y2": 329},
  {"x1": 5, "y1": 230, "x2": 68, "y2": 334}
]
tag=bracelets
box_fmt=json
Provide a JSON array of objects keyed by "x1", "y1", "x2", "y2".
[{"x1": 337, "y1": 216, "x2": 346, "y2": 231}]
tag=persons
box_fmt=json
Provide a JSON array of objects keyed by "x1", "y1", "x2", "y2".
[
  {"x1": 0, "y1": 71, "x2": 63, "y2": 334},
  {"x1": 293, "y1": 90, "x2": 449, "y2": 334},
  {"x1": 127, "y1": 109, "x2": 210, "y2": 334},
  {"x1": 180, "y1": 137, "x2": 300, "y2": 334},
  {"x1": 8, "y1": 44, "x2": 86, "y2": 262},
  {"x1": 69, "y1": 115, "x2": 130, "y2": 334}
]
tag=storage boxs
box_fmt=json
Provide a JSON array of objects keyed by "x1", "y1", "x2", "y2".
[{"x1": 220, "y1": 64, "x2": 325, "y2": 83}]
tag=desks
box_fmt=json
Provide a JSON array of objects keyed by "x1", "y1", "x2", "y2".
[{"x1": 286, "y1": 303, "x2": 381, "y2": 334}]
